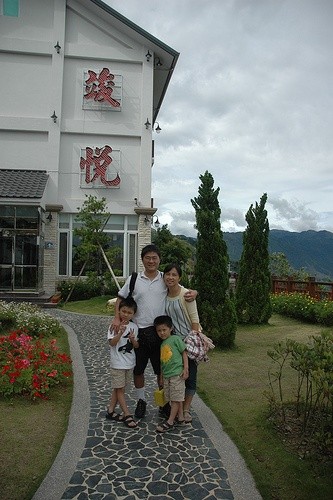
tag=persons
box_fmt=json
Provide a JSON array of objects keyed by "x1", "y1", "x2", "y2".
[
  {"x1": 109, "y1": 245, "x2": 176, "y2": 418},
  {"x1": 106, "y1": 298, "x2": 139, "y2": 428},
  {"x1": 163, "y1": 263, "x2": 203, "y2": 423},
  {"x1": 154, "y1": 316, "x2": 188, "y2": 432}
]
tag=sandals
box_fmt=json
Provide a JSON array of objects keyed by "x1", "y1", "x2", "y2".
[
  {"x1": 157, "y1": 421, "x2": 176, "y2": 432},
  {"x1": 105, "y1": 410, "x2": 123, "y2": 421},
  {"x1": 174, "y1": 418, "x2": 185, "y2": 426},
  {"x1": 183, "y1": 410, "x2": 193, "y2": 423},
  {"x1": 123, "y1": 416, "x2": 136, "y2": 428}
]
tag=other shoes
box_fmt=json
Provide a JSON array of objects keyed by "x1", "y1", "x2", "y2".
[
  {"x1": 158, "y1": 403, "x2": 173, "y2": 419},
  {"x1": 134, "y1": 399, "x2": 147, "y2": 417}
]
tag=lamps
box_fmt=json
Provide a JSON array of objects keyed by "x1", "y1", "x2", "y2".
[
  {"x1": 54, "y1": 42, "x2": 61, "y2": 54},
  {"x1": 36, "y1": 235, "x2": 41, "y2": 246},
  {"x1": 145, "y1": 118, "x2": 151, "y2": 129},
  {"x1": 51, "y1": 110, "x2": 58, "y2": 123},
  {"x1": 46, "y1": 211, "x2": 54, "y2": 222},
  {"x1": 155, "y1": 57, "x2": 163, "y2": 66},
  {"x1": 144, "y1": 214, "x2": 150, "y2": 225},
  {"x1": 152, "y1": 215, "x2": 161, "y2": 227},
  {"x1": 47, "y1": 241, "x2": 53, "y2": 249},
  {"x1": 153, "y1": 122, "x2": 162, "y2": 133},
  {"x1": 146, "y1": 50, "x2": 152, "y2": 61}
]
